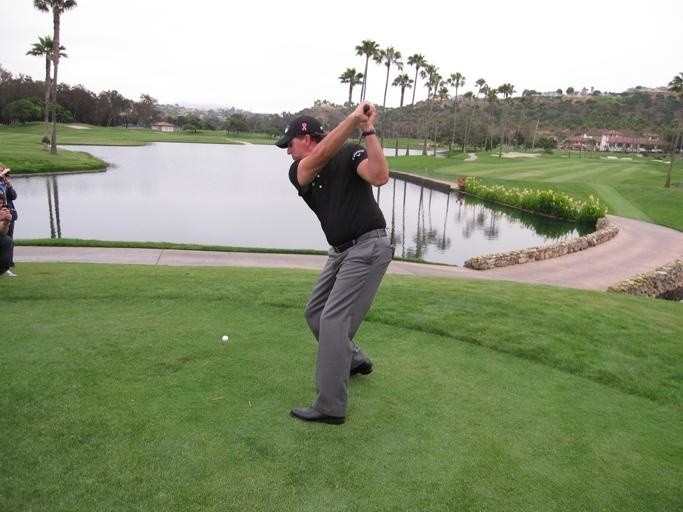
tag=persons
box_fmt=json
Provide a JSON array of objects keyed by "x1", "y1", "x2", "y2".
[
  {"x1": 274, "y1": 100, "x2": 394, "y2": 424},
  {"x1": 0, "y1": 163, "x2": 17, "y2": 267},
  {"x1": 0, "y1": 193, "x2": 17, "y2": 276}
]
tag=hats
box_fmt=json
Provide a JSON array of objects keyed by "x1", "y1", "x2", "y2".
[{"x1": 274, "y1": 115, "x2": 324, "y2": 148}]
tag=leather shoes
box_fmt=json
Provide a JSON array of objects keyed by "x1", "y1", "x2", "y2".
[
  {"x1": 349, "y1": 358, "x2": 373, "y2": 376},
  {"x1": 289, "y1": 406, "x2": 344, "y2": 425}
]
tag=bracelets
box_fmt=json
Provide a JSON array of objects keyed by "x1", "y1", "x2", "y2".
[
  {"x1": 4, "y1": 222, "x2": 10, "y2": 226},
  {"x1": 361, "y1": 130, "x2": 377, "y2": 138}
]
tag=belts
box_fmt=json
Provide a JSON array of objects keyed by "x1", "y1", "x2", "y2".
[{"x1": 333, "y1": 228, "x2": 386, "y2": 254}]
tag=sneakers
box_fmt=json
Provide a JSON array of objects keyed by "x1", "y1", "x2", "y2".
[{"x1": 3, "y1": 270, "x2": 17, "y2": 277}]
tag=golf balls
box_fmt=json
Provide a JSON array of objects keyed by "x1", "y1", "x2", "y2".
[{"x1": 222, "y1": 335, "x2": 228, "y2": 341}]
